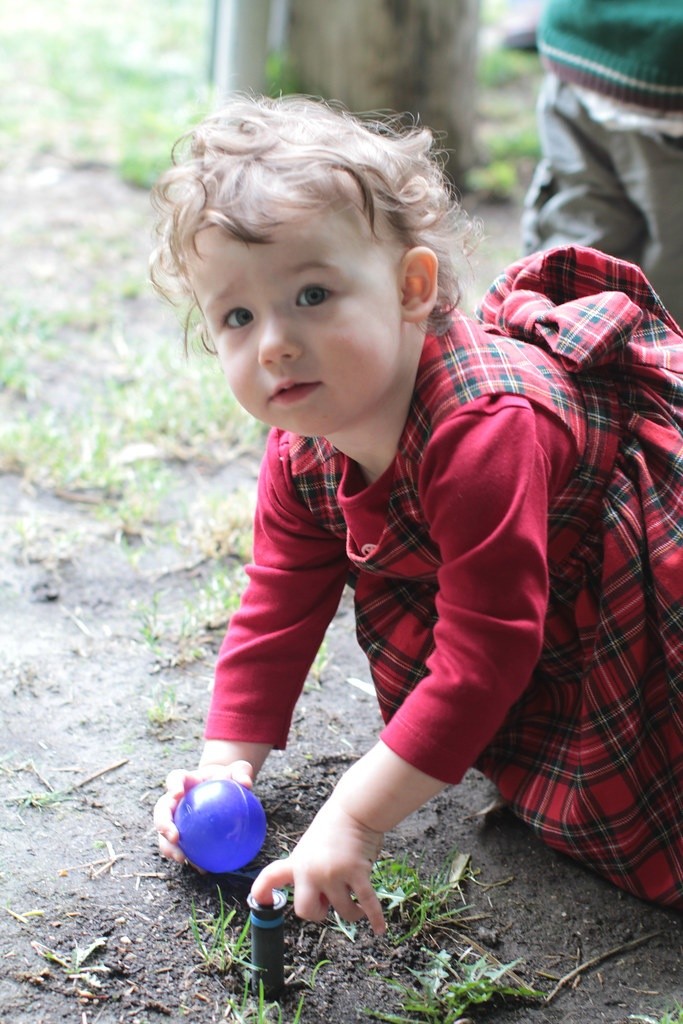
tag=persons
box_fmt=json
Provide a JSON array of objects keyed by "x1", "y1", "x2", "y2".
[
  {"x1": 517, "y1": 0, "x2": 682, "y2": 327},
  {"x1": 154, "y1": 97, "x2": 682, "y2": 935}
]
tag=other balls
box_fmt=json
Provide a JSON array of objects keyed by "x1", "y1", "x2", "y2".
[{"x1": 173, "y1": 777, "x2": 270, "y2": 876}]
